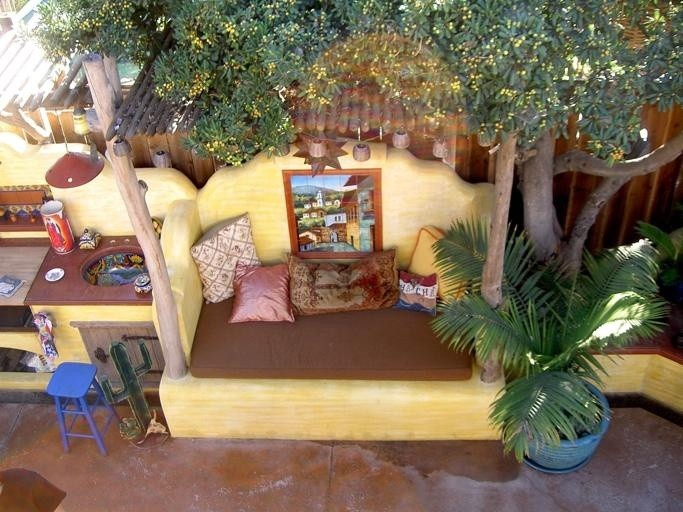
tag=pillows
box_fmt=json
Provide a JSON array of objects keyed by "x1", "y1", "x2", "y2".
[
  {"x1": 226, "y1": 262, "x2": 296, "y2": 322},
  {"x1": 392, "y1": 271, "x2": 437, "y2": 315},
  {"x1": 408, "y1": 227, "x2": 468, "y2": 308},
  {"x1": 286, "y1": 248, "x2": 397, "y2": 317},
  {"x1": 190, "y1": 211, "x2": 263, "y2": 304}
]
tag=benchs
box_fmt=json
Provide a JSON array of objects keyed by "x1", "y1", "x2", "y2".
[{"x1": 159, "y1": 137, "x2": 508, "y2": 442}]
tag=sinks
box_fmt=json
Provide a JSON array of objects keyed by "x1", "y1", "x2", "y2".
[{"x1": 78, "y1": 245, "x2": 148, "y2": 288}]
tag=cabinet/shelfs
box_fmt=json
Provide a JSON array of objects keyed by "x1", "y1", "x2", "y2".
[{"x1": 0, "y1": 245, "x2": 59, "y2": 403}]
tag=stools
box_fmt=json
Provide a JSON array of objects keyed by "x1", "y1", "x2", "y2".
[{"x1": 46, "y1": 363, "x2": 121, "y2": 456}]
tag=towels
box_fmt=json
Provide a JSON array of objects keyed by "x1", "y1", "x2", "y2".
[{"x1": 0, "y1": 275, "x2": 27, "y2": 299}]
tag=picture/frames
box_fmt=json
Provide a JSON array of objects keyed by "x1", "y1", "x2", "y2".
[{"x1": 282, "y1": 168, "x2": 382, "y2": 260}]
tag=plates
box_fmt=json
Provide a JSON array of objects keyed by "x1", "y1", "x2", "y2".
[{"x1": 44, "y1": 267, "x2": 65, "y2": 282}]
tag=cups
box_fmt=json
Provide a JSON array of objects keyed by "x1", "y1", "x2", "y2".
[{"x1": 134, "y1": 274, "x2": 153, "y2": 295}]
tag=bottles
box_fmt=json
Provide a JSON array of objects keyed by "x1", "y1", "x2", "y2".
[{"x1": 40, "y1": 200, "x2": 76, "y2": 256}]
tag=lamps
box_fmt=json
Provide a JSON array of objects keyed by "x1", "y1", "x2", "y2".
[{"x1": 45, "y1": 105, "x2": 104, "y2": 189}]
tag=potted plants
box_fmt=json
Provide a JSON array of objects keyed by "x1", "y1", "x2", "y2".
[{"x1": 428, "y1": 207, "x2": 678, "y2": 476}]
